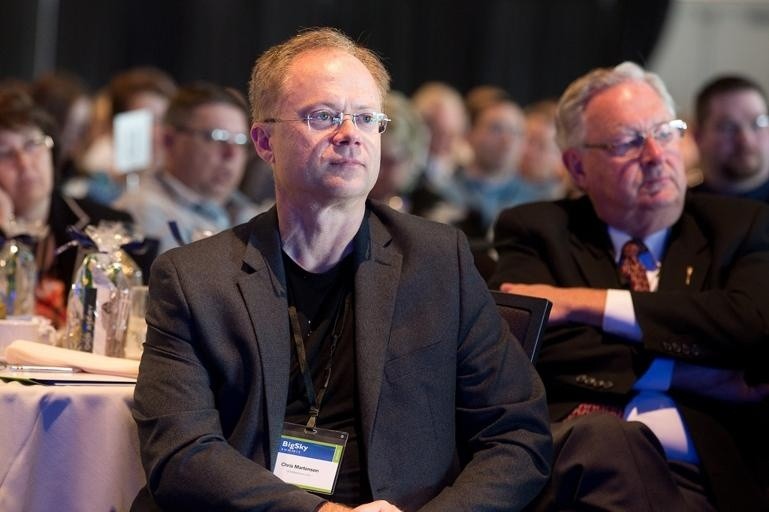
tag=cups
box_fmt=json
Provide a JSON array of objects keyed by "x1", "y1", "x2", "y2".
[{"x1": 123, "y1": 285, "x2": 148, "y2": 361}]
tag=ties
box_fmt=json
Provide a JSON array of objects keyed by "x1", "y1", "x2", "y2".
[{"x1": 617, "y1": 241, "x2": 648, "y2": 294}]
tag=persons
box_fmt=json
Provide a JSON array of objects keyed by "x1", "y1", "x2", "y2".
[
  {"x1": 131, "y1": 26, "x2": 553, "y2": 512},
  {"x1": 689, "y1": 76, "x2": 768, "y2": 202},
  {"x1": 1, "y1": 67, "x2": 703, "y2": 331},
  {"x1": 492, "y1": 62, "x2": 769, "y2": 511}
]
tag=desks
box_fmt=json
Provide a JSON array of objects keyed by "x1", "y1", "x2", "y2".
[{"x1": 0, "y1": 316, "x2": 149, "y2": 512}]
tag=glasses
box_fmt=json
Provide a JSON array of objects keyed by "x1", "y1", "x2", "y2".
[
  {"x1": 172, "y1": 124, "x2": 249, "y2": 148},
  {"x1": 22, "y1": 135, "x2": 54, "y2": 155},
  {"x1": 702, "y1": 112, "x2": 767, "y2": 134},
  {"x1": 260, "y1": 109, "x2": 392, "y2": 134},
  {"x1": 582, "y1": 120, "x2": 686, "y2": 157}
]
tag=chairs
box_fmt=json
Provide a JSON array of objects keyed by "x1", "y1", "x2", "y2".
[{"x1": 489, "y1": 288, "x2": 552, "y2": 370}]
